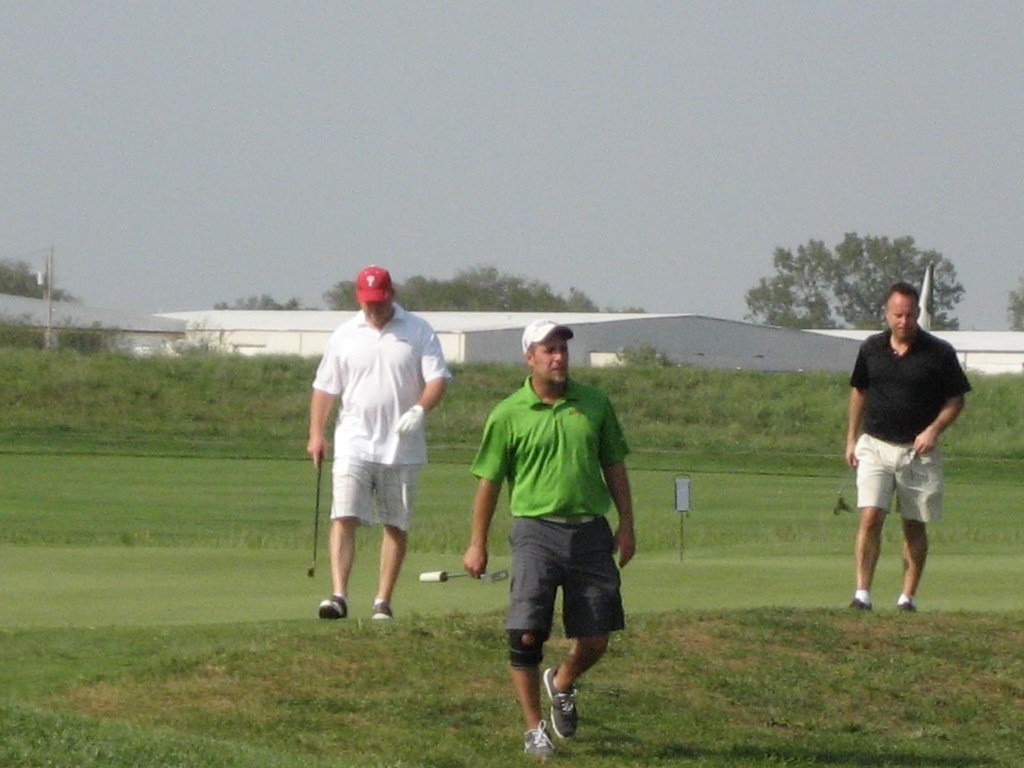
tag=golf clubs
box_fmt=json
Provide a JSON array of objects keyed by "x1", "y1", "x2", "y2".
[
  {"x1": 416, "y1": 567, "x2": 510, "y2": 585},
  {"x1": 837, "y1": 465, "x2": 859, "y2": 513},
  {"x1": 306, "y1": 456, "x2": 322, "y2": 579}
]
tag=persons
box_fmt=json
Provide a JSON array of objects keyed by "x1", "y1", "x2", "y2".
[
  {"x1": 305, "y1": 263, "x2": 456, "y2": 621},
  {"x1": 840, "y1": 279, "x2": 977, "y2": 611},
  {"x1": 462, "y1": 316, "x2": 641, "y2": 763}
]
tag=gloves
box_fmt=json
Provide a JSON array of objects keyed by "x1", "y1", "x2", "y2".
[{"x1": 396, "y1": 404, "x2": 425, "y2": 436}]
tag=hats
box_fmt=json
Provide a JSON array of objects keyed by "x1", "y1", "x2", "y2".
[
  {"x1": 522, "y1": 321, "x2": 573, "y2": 355},
  {"x1": 356, "y1": 266, "x2": 392, "y2": 302}
]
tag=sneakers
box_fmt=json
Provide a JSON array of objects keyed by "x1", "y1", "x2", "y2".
[
  {"x1": 524, "y1": 719, "x2": 555, "y2": 759},
  {"x1": 544, "y1": 666, "x2": 577, "y2": 738},
  {"x1": 372, "y1": 601, "x2": 393, "y2": 619},
  {"x1": 319, "y1": 594, "x2": 347, "y2": 619}
]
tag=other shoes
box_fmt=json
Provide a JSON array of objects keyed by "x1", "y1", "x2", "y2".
[
  {"x1": 897, "y1": 602, "x2": 917, "y2": 611},
  {"x1": 848, "y1": 597, "x2": 872, "y2": 611}
]
